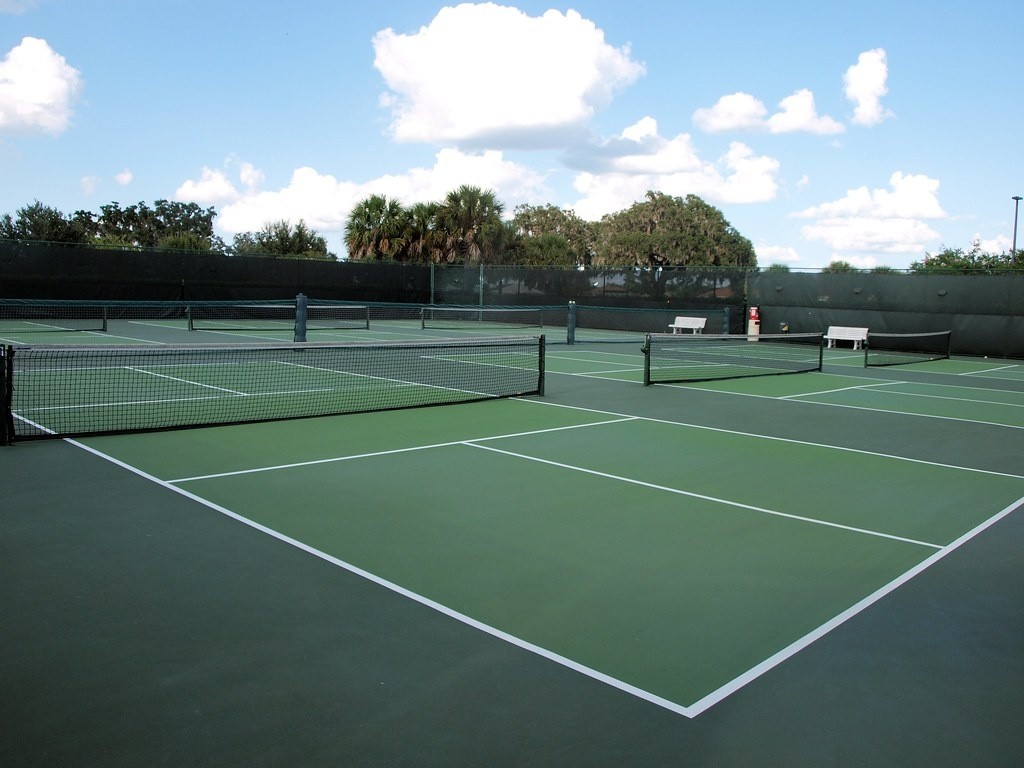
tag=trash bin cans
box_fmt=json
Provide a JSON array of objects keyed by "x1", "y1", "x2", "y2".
[{"x1": 747, "y1": 320, "x2": 760, "y2": 342}]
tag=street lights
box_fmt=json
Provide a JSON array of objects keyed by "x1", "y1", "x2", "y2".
[{"x1": 1011, "y1": 196, "x2": 1023, "y2": 263}]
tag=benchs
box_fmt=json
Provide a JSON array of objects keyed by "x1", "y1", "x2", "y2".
[
  {"x1": 668, "y1": 316, "x2": 708, "y2": 335},
  {"x1": 823, "y1": 326, "x2": 869, "y2": 351}
]
